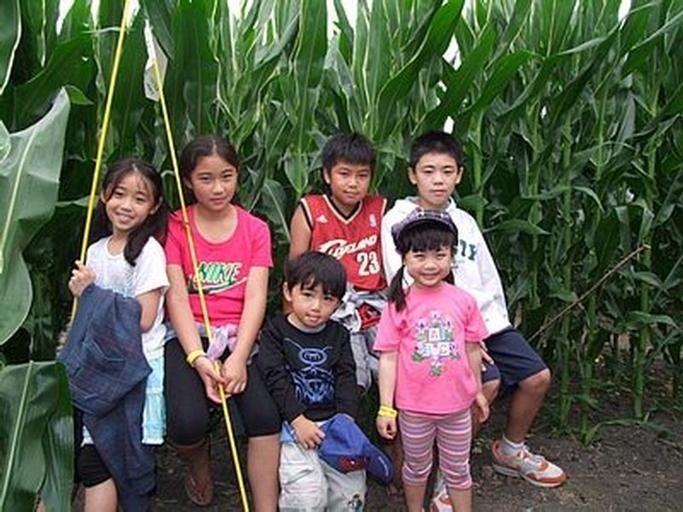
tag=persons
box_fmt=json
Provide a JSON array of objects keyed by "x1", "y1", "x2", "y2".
[
  {"x1": 375, "y1": 130, "x2": 568, "y2": 511},
  {"x1": 152, "y1": 135, "x2": 282, "y2": 511},
  {"x1": 288, "y1": 130, "x2": 387, "y2": 398},
  {"x1": 68, "y1": 158, "x2": 173, "y2": 511},
  {"x1": 370, "y1": 207, "x2": 489, "y2": 512},
  {"x1": 256, "y1": 250, "x2": 367, "y2": 512}
]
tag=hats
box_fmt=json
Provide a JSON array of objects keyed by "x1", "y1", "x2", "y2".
[
  {"x1": 391, "y1": 206, "x2": 458, "y2": 246},
  {"x1": 314, "y1": 412, "x2": 396, "y2": 488}
]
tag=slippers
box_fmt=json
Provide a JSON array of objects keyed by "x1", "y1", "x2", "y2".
[{"x1": 181, "y1": 465, "x2": 218, "y2": 505}]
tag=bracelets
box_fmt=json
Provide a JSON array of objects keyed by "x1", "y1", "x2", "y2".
[
  {"x1": 376, "y1": 404, "x2": 397, "y2": 416},
  {"x1": 186, "y1": 349, "x2": 207, "y2": 366}
]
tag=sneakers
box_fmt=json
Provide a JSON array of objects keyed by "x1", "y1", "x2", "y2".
[{"x1": 490, "y1": 435, "x2": 567, "y2": 488}]
